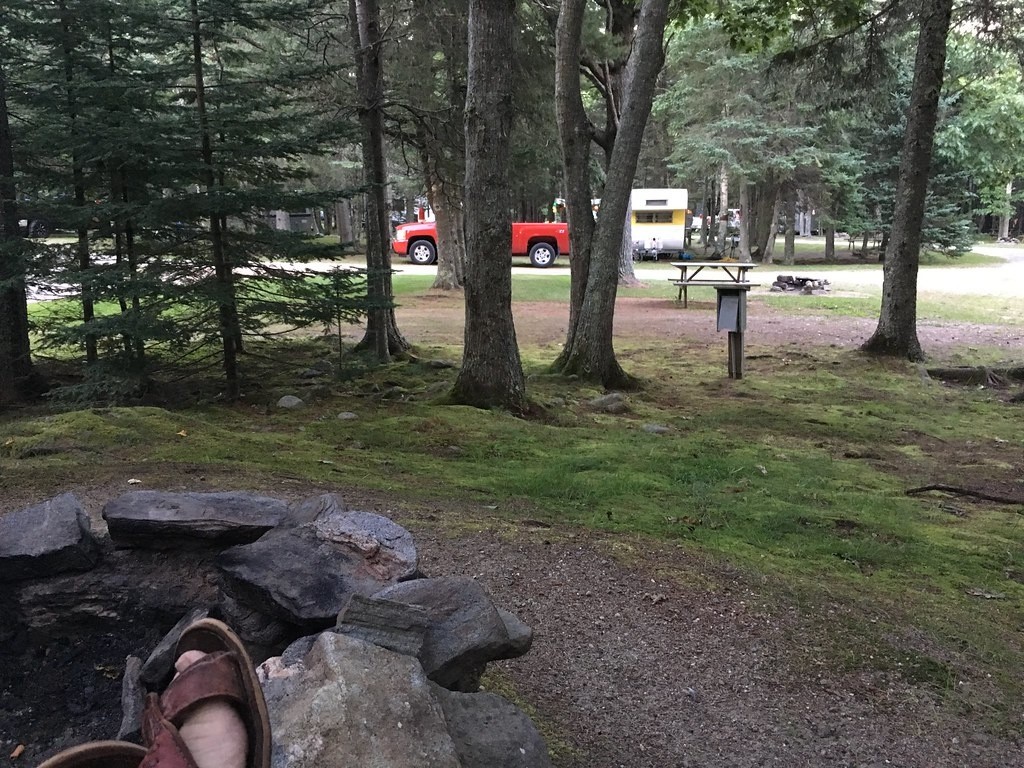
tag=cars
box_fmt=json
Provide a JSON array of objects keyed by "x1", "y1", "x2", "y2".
[{"x1": 691, "y1": 208, "x2": 741, "y2": 234}]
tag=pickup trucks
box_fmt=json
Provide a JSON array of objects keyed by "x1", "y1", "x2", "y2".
[{"x1": 392, "y1": 216, "x2": 569, "y2": 268}]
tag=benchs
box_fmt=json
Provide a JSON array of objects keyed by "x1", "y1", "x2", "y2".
[
  {"x1": 668, "y1": 278, "x2": 761, "y2": 290},
  {"x1": 845, "y1": 238, "x2": 882, "y2": 242}
]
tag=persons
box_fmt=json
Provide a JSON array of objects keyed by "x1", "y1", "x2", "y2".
[
  {"x1": 592, "y1": 205, "x2": 598, "y2": 222},
  {"x1": 39, "y1": 617, "x2": 272, "y2": 768},
  {"x1": 651, "y1": 238, "x2": 658, "y2": 261},
  {"x1": 632, "y1": 240, "x2": 642, "y2": 264},
  {"x1": 559, "y1": 204, "x2": 566, "y2": 223}
]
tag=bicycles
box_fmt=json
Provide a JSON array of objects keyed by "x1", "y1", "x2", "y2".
[{"x1": 632, "y1": 241, "x2": 644, "y2": 264}]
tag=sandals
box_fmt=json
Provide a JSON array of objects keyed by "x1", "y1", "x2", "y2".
[{"x1": 140, "y1": 617, "x2": 271, "y2": 768}]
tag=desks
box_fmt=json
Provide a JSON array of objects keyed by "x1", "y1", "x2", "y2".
[
  {"x1": 671, "y1": 262, "x2": 759, "y2": 308},
  {"x1": 848, "y1": 233, "x2": 882, "y2": 250}
]
